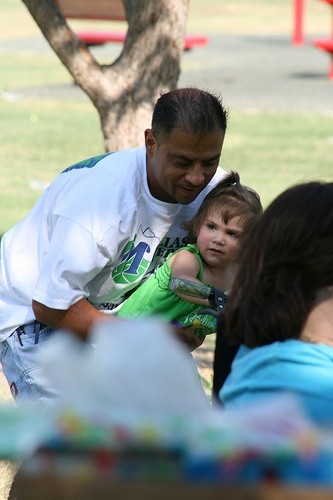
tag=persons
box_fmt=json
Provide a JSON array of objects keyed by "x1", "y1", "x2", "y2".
[
  {"x1": 1, "y1": 87, "x2": 240, "y2": 422},
  {"x1": 211, "y1": 181, "x2": 333, "y2": 455},
  {"x1": 117, "y1": 172, "x2": 258, "y2": 359}
]
